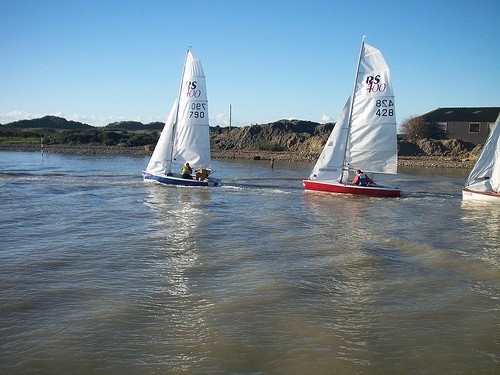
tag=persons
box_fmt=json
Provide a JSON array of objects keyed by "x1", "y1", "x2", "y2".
[
  {"x1": 352, "y1": 169, "x2": 373, "y2": 186},
  {"x1": 181, "y1": 162, "x2": 193, "y2": 180}
]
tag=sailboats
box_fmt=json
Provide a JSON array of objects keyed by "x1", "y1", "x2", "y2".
[
  {"x1": 303, "y1": 34, "x2": 402, "y2": 197},
  {"x1": 462, "y1": 107, "x2": 500, "y2": 204},
  {"x1": 142, "y1": 44, "x2": 222, "y2": 188}
]
